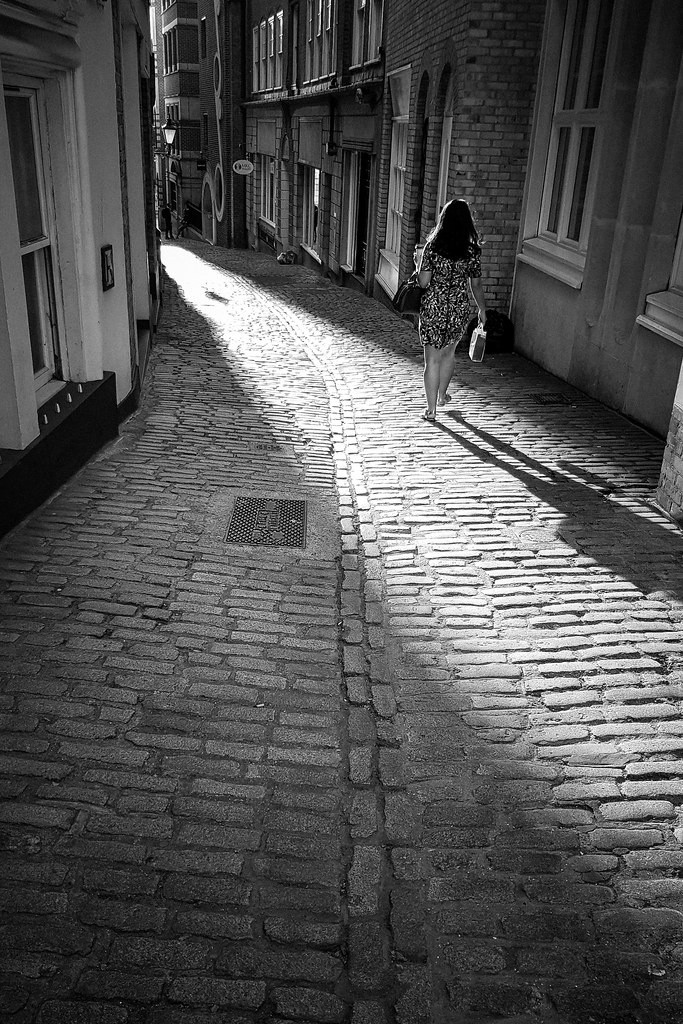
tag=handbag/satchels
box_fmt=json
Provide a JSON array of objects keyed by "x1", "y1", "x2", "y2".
[
  {"x1": 468, "y1": 322, "x2": 487, "y2": 362},
  {"x1": 393, "y1": 271, "x2": 425, "y2": 314}
]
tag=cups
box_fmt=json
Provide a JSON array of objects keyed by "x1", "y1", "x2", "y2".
[{"x1": 415, "y1": 245, "x2": 424, "y2": 257}]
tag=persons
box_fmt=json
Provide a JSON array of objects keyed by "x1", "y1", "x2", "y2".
[
  {"x1": 175, "y1": 208, "x2": 188, "y2": 239},
  {"x1": 164, "y1": 203, "x2": 173, "y2": 240},
  {"x1": 412, "y1": 200, "x2": 486, "y2": 421}
]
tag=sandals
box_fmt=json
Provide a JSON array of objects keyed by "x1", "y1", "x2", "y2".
[
  {"x1": 436, "y1": 394, "x2": 451, "y2": 407},
  {"x1": 421, "y1": 410, "x2": 436, "y2": 422}
]
